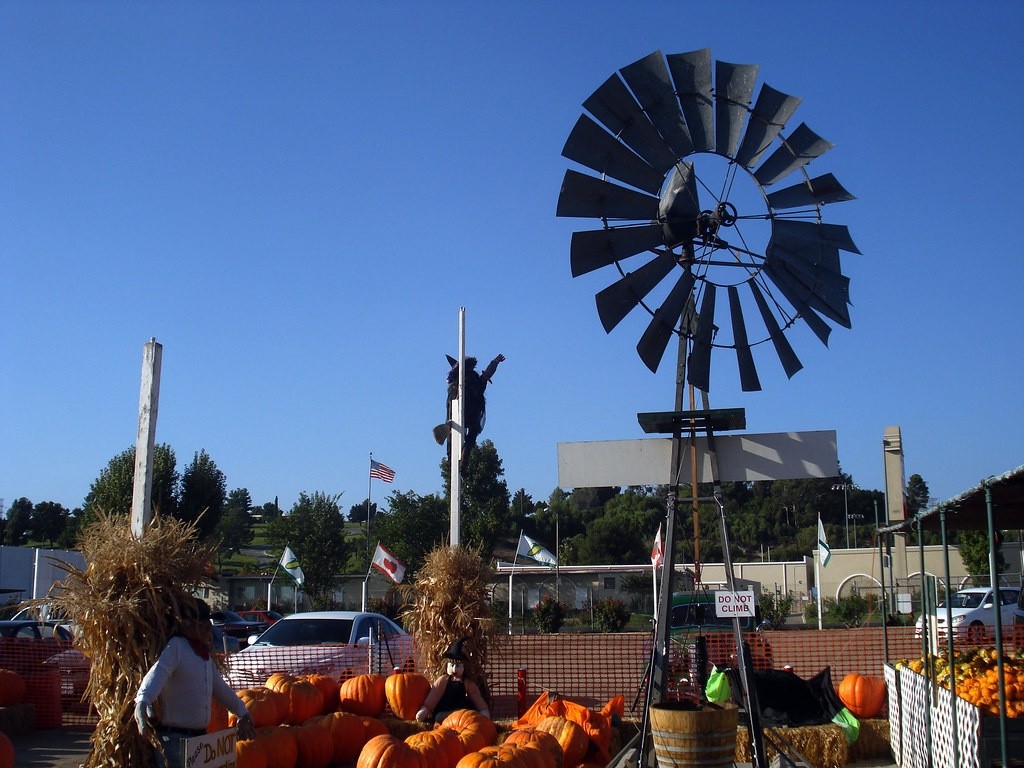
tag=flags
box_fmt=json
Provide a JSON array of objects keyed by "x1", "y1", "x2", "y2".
[
  {"x1": 650, "y1": 525, "x2": 664, "y2": 568},
  {"x1": 278, "y1": 546, "x2": 305, "y2": 588},
  {"x1": 517, "y1": 535, "x2": 558, "y2": 565},
  {"x1": 818, "y1": 519, "x2": 830, "y2": 566},
  {"x1": 370, "y1": 459, "x2": 396, "y2": 483},
  {"x1": 371, "y1": 543, "x2": 408, "y2": 585}
]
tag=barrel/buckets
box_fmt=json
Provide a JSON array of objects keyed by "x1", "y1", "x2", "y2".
[{"x1": 649, "y1": 700, "x2": 738, "y2": 768}]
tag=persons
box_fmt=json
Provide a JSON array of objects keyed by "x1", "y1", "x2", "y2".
[
  {"x1": 415, "y1": 633, "x2": 491, "y2": 724},
  {"x1": 132, "y1": 596, "x2": 255, "y2": 768}
]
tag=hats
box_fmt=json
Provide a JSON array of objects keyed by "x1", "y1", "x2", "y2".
[{"x1": 179, "y1": 599, "x2": 225, "y2": 623}]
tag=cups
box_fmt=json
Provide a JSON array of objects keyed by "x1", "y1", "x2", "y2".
[
  {"x1": 237, "y1": 638, "x2": 248, "y2": 650},
  {"x1": 223, "y1": 636, "x2": 237, "y2": 655}
]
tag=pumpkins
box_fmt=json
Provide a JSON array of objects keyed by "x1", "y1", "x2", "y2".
[
  {"x1": 0, "y1": 731, "x2": 15, "y2": 768},
  {"x1": 0, "y1": 668, "x2": 24, "y2": 706},
  {"x1": 837, "y1": 673, "x2": 886, "y2": 719},
  {"x1": 894, "y1": 644, "x2": 1024, "y2": 719},
  {"x1": 209, "y1": 671, "x2": 588, "y2": 768}
]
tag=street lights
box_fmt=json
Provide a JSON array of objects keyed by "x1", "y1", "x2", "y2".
[
  {"x1": 847, "y1": 513, "x2": 864, "y2": 548},
  {"x1": 831, "y1": 482, "x2": 860, "y2": 549}
]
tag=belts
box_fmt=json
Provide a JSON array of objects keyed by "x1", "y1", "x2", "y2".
[{"x1": 161, "y1": 726, "x2": 208, "y2": 737}]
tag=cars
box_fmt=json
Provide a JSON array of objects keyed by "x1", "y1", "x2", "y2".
[
  {"x1": 670, "y1": 588, "x2": 772, "y2": 644},
  {"x1": 220, "y1": 610, "x2": 418, "y2": 694},
  {"x1": 209, "y1": 608, "x2": 285, "y2": 669},
  {"x1": 915, "y1": 584, "x2": 1023, "y2": 644},
  {"x1": 0, "y1": 603, "x2": 92, "y2": 710}
]
